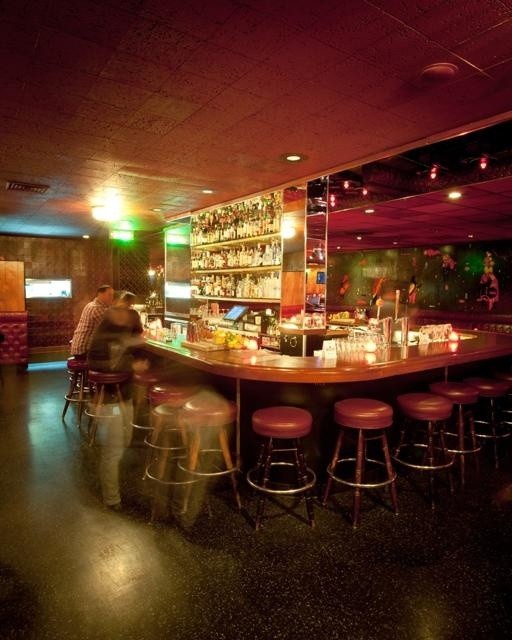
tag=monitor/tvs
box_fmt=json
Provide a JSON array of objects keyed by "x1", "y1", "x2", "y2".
[
  {"x1": 223, "y1": 305, "x2": 249, "y2": 322},
  {"x1": 317, "y1": 272, "x2": 325, "y2": 284}
]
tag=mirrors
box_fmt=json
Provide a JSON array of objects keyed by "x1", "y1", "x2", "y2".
[
  {"x1": 164, "y1": 215, "x2": 191, "y2": 319},
  {"x1": 280, "y1": 175, "x2": 327, "y2": 330}
]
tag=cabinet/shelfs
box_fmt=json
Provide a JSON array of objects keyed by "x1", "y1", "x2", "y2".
[{"x1": 193, "y1": 189, "x2": 283, "y2": 335}]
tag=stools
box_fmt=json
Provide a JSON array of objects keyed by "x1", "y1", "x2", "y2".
[
  {"x1": 141, "y1": 381, "x2": 205, "y2": 498},
  {"x1": 321, "y1": 399, "x2": 400, "y2": 531},
  {"x1": 245, "y1": 406, "x2": 317, "y2": 531},
  {"x1": 177, "y1": 397, "x2": 245, "y2": 515},
  {"x1": 63, "y1": 374, "x2": 170, "y2": 453},
  {"x1": 388, "y1": 373, "x2": 511, "y2": 510}
]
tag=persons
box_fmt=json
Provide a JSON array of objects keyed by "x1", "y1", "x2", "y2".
[
  {"x1": 82, "y1": 296, "x2": 151, "y2": 514},
  {"x1": 87, "y1": 291, "x2": 143, "y2": 405},
  {"x1": 70, "y1": 284, "x2": 115, "y2": 361}
]
